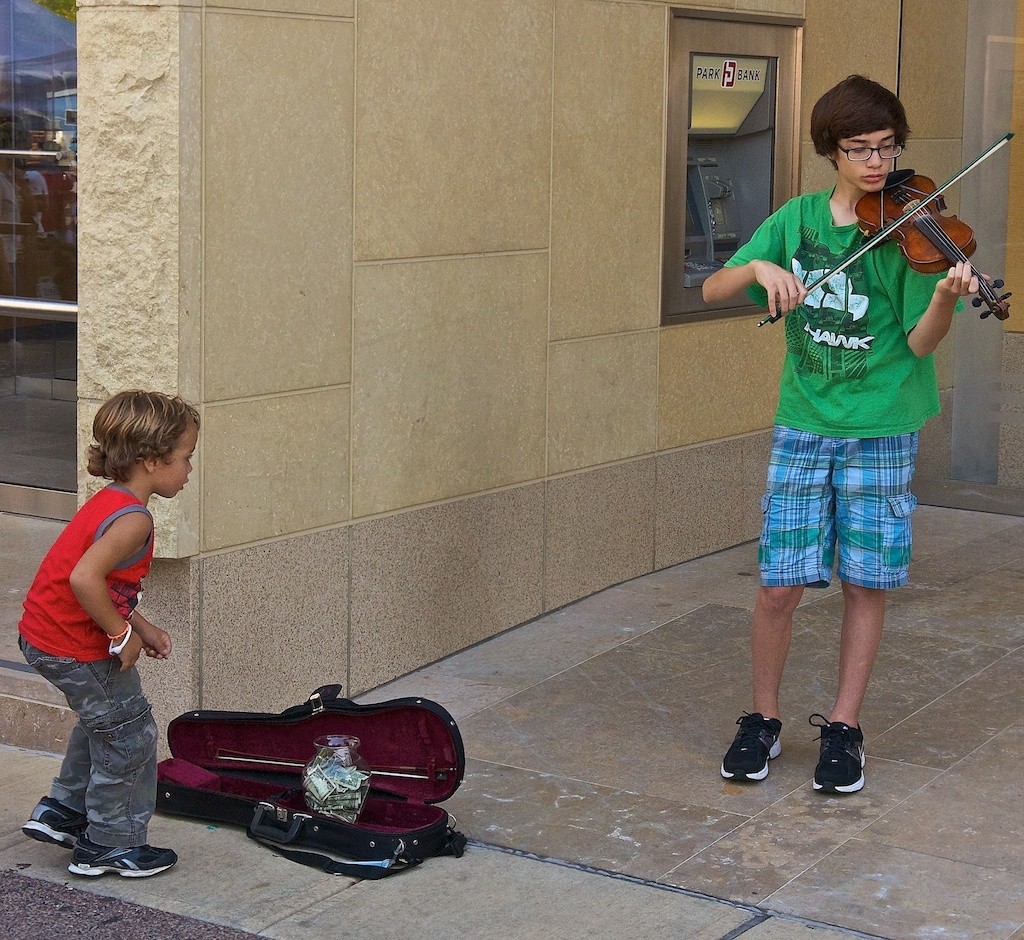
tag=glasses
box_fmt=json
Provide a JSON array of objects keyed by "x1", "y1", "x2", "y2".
[{"x1": 837, "y1": 143, "x2": 904, "y2": 161}]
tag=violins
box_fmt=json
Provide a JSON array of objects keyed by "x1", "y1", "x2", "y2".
[{"x1": 855, "y1": 174, "x2": 1013, "y2": 320}]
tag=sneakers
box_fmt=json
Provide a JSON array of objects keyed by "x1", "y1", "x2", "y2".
[
  {"x1": 66, "y1": 832, "x2": 179, "y2": 878},
  {"x1": 720, "y1": 710, "x2": 782, "y2": 781},
  {"x1": 809, "y1": 713, "x2": 867, "y2": 794},
  {"x1": 20, "y1": 796, "x2": 89, "y2": 849}
]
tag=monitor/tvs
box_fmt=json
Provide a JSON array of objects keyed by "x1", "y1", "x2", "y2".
[{"x1": 686, "y1": 203, "x2": 694, "y2": 225}]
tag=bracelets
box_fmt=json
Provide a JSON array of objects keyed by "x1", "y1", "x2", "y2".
[
  {"x1": 109, "y1": 624, "x2": 132, "y2": 655},
  {"x1": 107, "y1": 620, "x2": 129, "y2": 639}
]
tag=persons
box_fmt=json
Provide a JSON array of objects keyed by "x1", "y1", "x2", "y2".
[
  {"x1": 17, "y1": 390, "x2": 202, "y2": 878},
  {"x1": 701, "y1": 75, "x2": 989, "y2": 796},
  {"x1": 0, "y1": 113, "x2": 77, "y2": 341}
]
